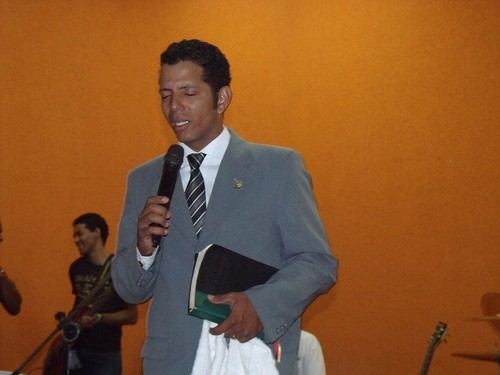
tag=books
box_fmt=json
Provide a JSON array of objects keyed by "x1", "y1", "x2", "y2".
[{"x1": 187, "y1": 243, "x2": 282, "y2": 342}]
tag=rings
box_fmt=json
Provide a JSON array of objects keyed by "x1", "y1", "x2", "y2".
[{"x1": 232, "y1": 334, "x2": 235, "y2": 340}]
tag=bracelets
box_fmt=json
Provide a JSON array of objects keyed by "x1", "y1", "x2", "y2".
[{"x1": 96, "y1": 314, "x2": 103, "y2": 324}]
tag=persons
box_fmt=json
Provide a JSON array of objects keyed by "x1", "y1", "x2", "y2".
[
  {"x1": 56, "y1": 212, "x2": 139, "y2": 375},
  {"x1": 295, "y1": 326, "x2": 326, "y2": 374},
  {"x1": 0, "y1": 224, "x2": 21, "y2": 317},
  {"x1": 111, "y1": 37, "x2": 341, "y2": 375}
]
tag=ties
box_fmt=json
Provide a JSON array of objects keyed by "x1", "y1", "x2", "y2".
[{"x1": 184, "y1": 154, "x2": 206, "y2": 244}]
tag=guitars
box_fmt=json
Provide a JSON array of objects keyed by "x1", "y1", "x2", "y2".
[
  {"x1": 43, "y1": 282, "x2": 116, "y2": 375},
  {"x1": 418, "y1": 321, "x2": 450, "y2": 374}
]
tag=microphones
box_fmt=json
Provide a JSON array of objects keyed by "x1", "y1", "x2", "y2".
[{"x1": 149, "y1": 145, "x2": 185, "y2": 245}]
